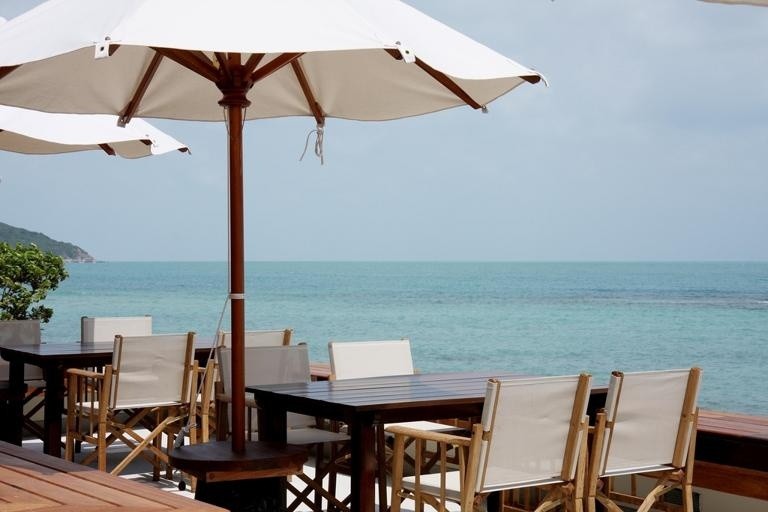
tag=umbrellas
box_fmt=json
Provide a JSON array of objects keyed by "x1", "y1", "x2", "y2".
[
  {"x1": 0, "y1": 12, "x2": 192, "y2": 164},
  {"x1": 0, "y1": 1, "x2": 550, "y2": 459}
]
